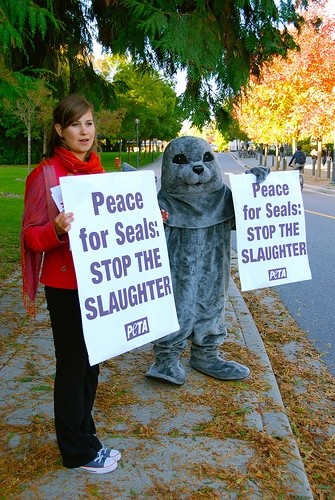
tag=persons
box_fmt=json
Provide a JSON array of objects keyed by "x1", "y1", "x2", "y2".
[
  {"x1": 311, "y1": 146, "x2": 333, "y2": 166},
  {"x1": 288, "y1": 146, "x2": 307, "y2": 185},
  {"x1": 20, "y1": 94, "x2": 169, "y2": 473},
  {"x1": 237, "y1": 142, "x2": 257, "y2": 159}
]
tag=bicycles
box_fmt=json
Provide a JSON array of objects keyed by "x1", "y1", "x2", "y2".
[{"x1": 290, "y1": 165, "x2": 304, "y2": 191}]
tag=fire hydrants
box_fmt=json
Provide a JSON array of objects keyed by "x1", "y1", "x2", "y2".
[{"x1": 114, "y1": 157, "x2": 120, "y2": 169}]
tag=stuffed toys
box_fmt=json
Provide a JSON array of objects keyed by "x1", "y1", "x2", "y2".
[{"x1": 123, "y1": 135, "x2": 270, "y2": 385}]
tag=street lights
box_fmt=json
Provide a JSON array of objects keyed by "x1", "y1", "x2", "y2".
[{"x1": 135, "y1": 119, "x2": 140, "y2": 170}]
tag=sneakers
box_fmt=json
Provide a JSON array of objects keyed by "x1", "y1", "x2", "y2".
[
  {"x1": 74, "y1": 451, "x2": 117, "y2": 474},
  {"x1": 97, "y1": 445, "x2": 121, "y2": 462}
]
